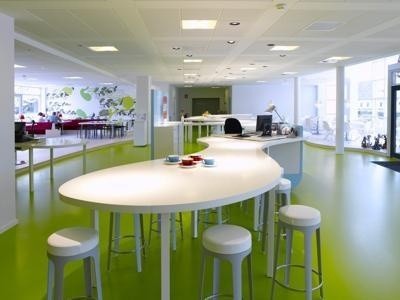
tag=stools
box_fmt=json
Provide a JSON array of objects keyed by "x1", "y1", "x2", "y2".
[
  {"x1": 271, "y1": 204, "x2": 324, "y2": 300},
  {"x1": 198, "y1": 204, "x2": 232, "y2": 226},
  {"x1": 148, "y1": 213, "x2": 185, "y2": 246},
  {"x1": 261, "y1": 166, "x2": 284, "y2": 231},
  {"x1": 107, "y1": 211, "x2": 147, "y2": 269},
  {"x1": 200, "y1": 224, "x2": 254, "y2": 300},
  {"x1": 46, "y1": 227, "x2": 102, "y2": 300},
  {"x1": 256, "y1": 178, "x2": 294, "y2": 254}
]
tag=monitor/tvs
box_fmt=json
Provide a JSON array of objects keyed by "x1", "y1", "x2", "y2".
[
  {"x1": 256, "y1": 115, "x2": 272, "y2": 137},
  {"x1": 390, "y1": 85, "x2": 400, "y2": 153}
]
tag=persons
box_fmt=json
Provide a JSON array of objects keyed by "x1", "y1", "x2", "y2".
[
  {"x1": 36, "y1": 112, "x2": 46, "y2": 122},
  {"x1": 18, "y1": 113, "x2": 26, "y2": 122},
  {"x1": 47, "y1": 111, "x2": 62, "y2": 122}
]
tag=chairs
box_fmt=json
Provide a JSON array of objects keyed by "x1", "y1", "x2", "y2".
[{"x1": 225, "y1": 118, "x2": 242, "y2": 134}]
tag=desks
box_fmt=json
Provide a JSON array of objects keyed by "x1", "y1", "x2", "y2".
[
  {"x1": 13, "y1": 137, "x2": 89, "y2": 194},
  {"x1": 182, "y1": 114, "x2": 251, "y2": 143},
  {"x1": 78, "y1": 121, "x2": 115, "y2": 139},
  {"x1": 58, "y1": 120, "x2": 310, "y2": 300}
]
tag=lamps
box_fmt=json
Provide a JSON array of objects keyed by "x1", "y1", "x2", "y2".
[{"x1": 264, "y1": 102, "x2": 286, "y2": 122}]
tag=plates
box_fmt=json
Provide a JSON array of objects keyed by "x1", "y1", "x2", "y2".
[
  {"x1": 178, "y1": 163, "x2": 197, "y2": 167},
  {"x1": 163, "y1": 160, "x2": 180, "y2": 162},
  {"x1": 194, "y1": 160, "x2": 203, "y2": 163},
  {"x1": 204, "y1": 164, "x2": 216, "y2": 167}
]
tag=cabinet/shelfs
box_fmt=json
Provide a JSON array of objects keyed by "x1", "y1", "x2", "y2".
[{"x1": 154, "y1": 121, "x2": 184, "y2": 157}]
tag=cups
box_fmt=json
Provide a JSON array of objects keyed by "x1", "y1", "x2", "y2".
[
  {"x1": 182, "y1": 160, "x2": 193, "y2": 166},
  {"x1": 204, "y1": 158, "x2": 214, "y2": 165},
  {"x1": 166, "y1": 154, "x2": 179, "y2": 162},
  {"x1": 188, "y1": 155, "x2": 203, "y2": 161}
]
